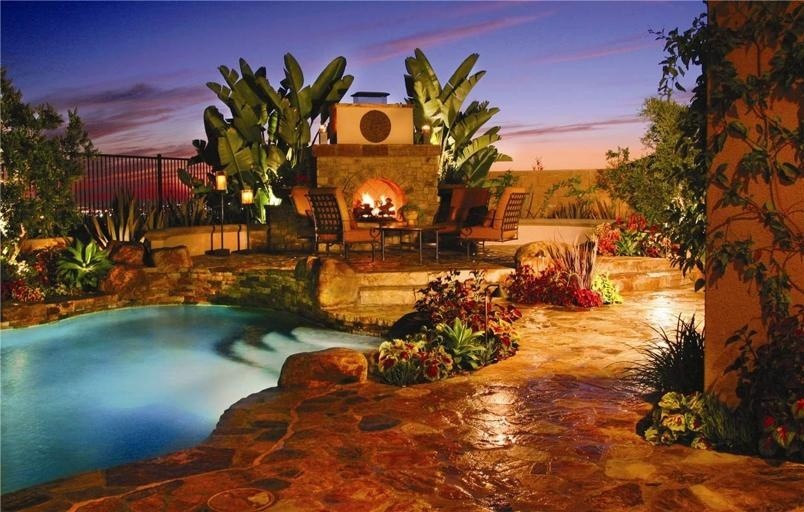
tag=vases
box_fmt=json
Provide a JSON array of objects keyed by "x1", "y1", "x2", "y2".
[{"x1": 437, "y1": 182, "x2": 467, "y2": 226}]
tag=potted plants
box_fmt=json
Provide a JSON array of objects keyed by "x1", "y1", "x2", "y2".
[{"x1": 399, "y1": 202, "x2": 426, "y2": 229}]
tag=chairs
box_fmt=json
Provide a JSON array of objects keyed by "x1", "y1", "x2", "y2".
[
  {"x1": 288, "y1": 186, "x2": 330, "y2": 258},
  {"x1": 425, "y1": 185, "x2": 490, "y2": 256},
  {"x1": 304, "y1": 185, "x2": 381, "y2": 264}
]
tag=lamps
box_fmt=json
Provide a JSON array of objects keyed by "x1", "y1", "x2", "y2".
[
  {"x1": 213, "y1": 170, "x2": 231, "y2": 257},
  {"x1": 240, "y1": 188, "x2": 255, "y2": 255}
]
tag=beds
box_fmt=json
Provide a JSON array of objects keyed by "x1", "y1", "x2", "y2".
[{"x1": 457, "y1": 183, "x2": 528, "y2": 261}]
